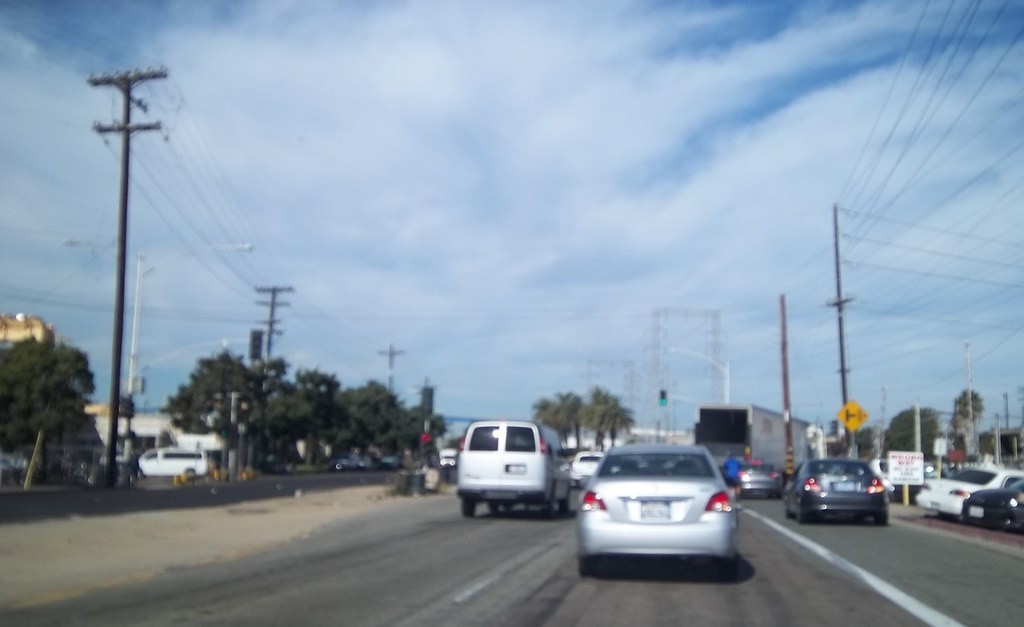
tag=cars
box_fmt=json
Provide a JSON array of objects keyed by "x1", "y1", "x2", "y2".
[
  {"x1": 736, "y1": 458, "x2": 784, "y2": 501},
  {"x1": 914, "y1": 460, "x2": 1024, "y2": 518},
  {"x1": 571, "y1": 452, "x2": 608, "y2": 488},
  {"x1": 785, "y1": 458, "x2": 889, "y2": 526},
  {"x1": 958, "y1": 480, "x2": 1024, "y2": 532},
  {"x1": 571, "y1": 443, "x2": 741, "y2": 573}
]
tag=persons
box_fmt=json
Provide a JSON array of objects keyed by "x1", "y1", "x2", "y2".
[{"x1": 723, "y1": 452, "x2": 742, "y2": 510}]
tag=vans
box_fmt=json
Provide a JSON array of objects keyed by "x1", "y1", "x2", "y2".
[
  {"x1": 457, "y1": 419, "x2": 570, "y2": 518},
  {"x1": 138, "y1": 446, "x2": 211, "y2": 482}
]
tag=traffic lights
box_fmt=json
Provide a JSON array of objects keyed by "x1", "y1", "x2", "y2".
[{"x1": 659, "y1": 389, "x2": 669, "y2": 410}]
